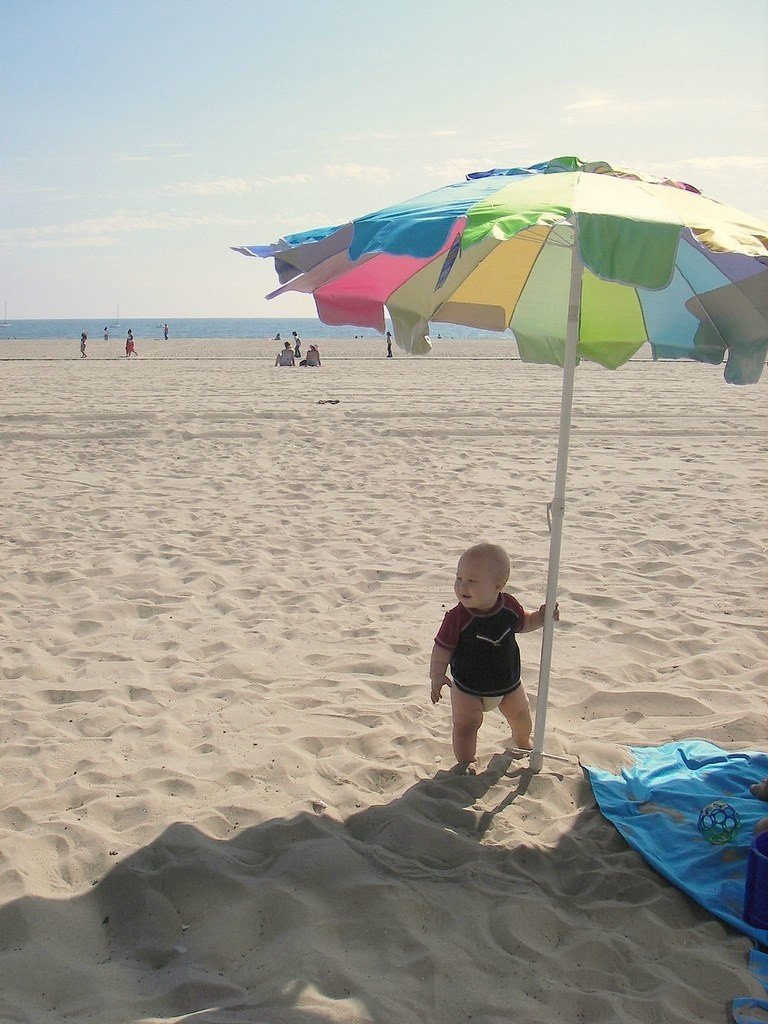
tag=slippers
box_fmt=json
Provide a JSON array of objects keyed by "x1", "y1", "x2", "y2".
[{"x1": 318, "y1": 400, "x2": 340, "y2": 404}]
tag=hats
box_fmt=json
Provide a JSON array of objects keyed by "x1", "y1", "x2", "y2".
[{"x1": 310, "y1": 343, "x2": 320, "y2": 353}]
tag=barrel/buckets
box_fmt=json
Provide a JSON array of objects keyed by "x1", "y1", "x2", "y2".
[{"x1": 743, "y1": 830, "x2": 768, "y2": 930}]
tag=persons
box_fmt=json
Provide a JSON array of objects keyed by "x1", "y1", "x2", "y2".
[
  {"x1": 104, "y1": 326, "x2": 109, "y2": 340},
  {"x1": 386, "y1": 332, "x2": 393, "y2": 357},
  {"x1": 164, "y1": 323, "x2": 169, "y2": 340},
  {"x1": 306, "y1": 343, "x2": 321, "y2": 367},
  {"x1": 275, "y1": 341, "x2": 295, "y2": 366},
  {"x1": 272, "y1": 333, "x2": 281, "y2": 340},
  {"x1": 125, "y1": 329, "x2": 138, "y2": 356},
  {"x1": 292, "y1": 331, "x2": 301, "y2": 357},
  {"x1": 80, "y1": 332, "x2": 87, "y2": 358},
  {"x1": 429, "y1": 543, "x2": 560, "y2": 775}
]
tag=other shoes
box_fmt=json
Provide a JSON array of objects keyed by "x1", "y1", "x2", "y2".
[{"x1": 387, "y1": 356, "x2": 392, "y2": 357}]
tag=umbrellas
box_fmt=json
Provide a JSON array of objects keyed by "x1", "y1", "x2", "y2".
[{"x1": 229, "y1": 158, "x2": 766, "y2": 779}]
